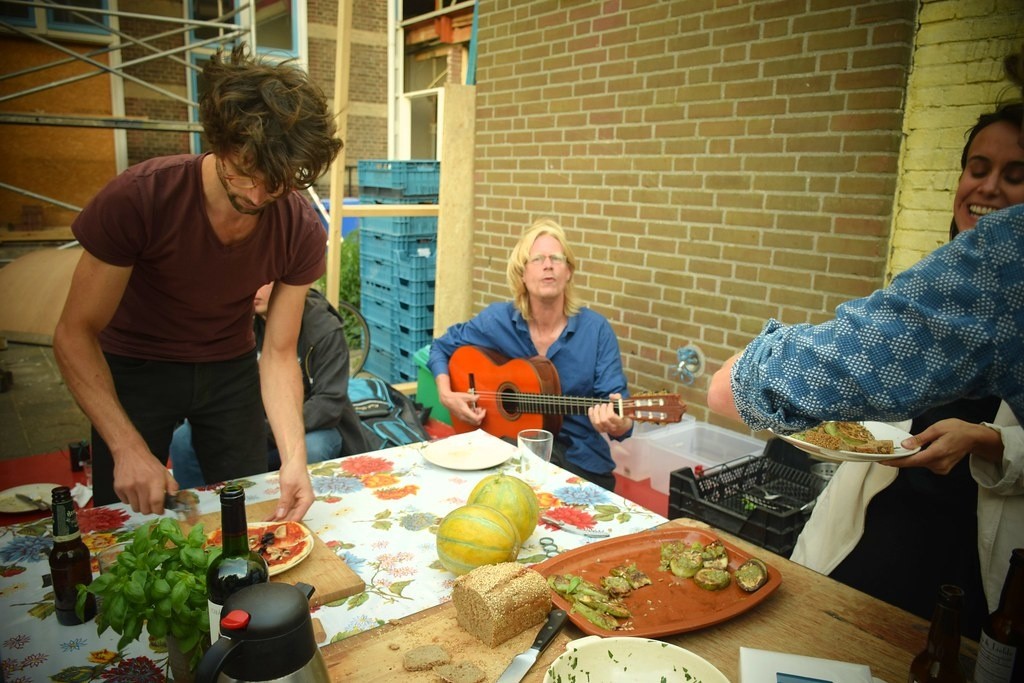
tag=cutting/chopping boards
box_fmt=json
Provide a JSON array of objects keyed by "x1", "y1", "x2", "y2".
[{"x1": 153, "y1": 495, "x2": 367, "y2": 614}]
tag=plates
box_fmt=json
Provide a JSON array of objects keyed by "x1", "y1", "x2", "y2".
[
  {"x1": 244, "y1": 520, "x2": 314, "y2": 575},
  {"x1": 766, "y1": 420, "x2": 921, "y2": 462},
  {"x1": 0, "y1": 482, "x2": 62, "y2": 513},
  {"x1": 532, "y1": 526, "x2": 783, "y2": 638},
  {"x1": 420, "y1": 436, "x2": 513, "y2": 470}
]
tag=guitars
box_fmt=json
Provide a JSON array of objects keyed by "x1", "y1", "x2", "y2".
[{"x1": 445, "y1": 344, "x2": 689, "y2": 439}]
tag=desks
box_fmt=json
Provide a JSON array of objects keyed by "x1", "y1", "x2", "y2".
[
  {"x1": 0, "y1": 429, "x2": 979, "y2": 683},
  {"x1": 0, "y1": 446, "x2": 172, "y2": 531}
]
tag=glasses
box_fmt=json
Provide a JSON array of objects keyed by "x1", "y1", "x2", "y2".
[
  {"x1": 527, "y1": 252, "x2": 568, "y2": 266},
  {"x1": 219, "y1": 154, "x2": 295, "y2": 199}
]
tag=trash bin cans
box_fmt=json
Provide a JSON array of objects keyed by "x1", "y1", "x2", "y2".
[{"x1": 313, "y1": 197, "x2": 360, "y2": 238}]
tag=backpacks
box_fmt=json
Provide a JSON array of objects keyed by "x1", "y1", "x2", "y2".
[{"x1": 345, "y1": 376, "x2": 426, "y2": 450}]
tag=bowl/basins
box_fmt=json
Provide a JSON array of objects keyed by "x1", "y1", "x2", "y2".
[{"x1": 543, "y1": 634, "x2": 731, "y2": 683}]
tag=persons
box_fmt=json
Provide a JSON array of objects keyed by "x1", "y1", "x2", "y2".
[
  {"x1": 706, "y1": 39, "x2": 1024, "y2": 437},
  {"x1": 52, "y1": 40, "x2": 344, "y2": 524},
  {"x1": 790, "y1": 101, "x2": 1024, "y2": 645},
  {"x1": 168, "y1": 278, "x2": 373, "y2": 493},
  {"x1": 427, "y1": 218, "x2": 635, "y2": 495}
]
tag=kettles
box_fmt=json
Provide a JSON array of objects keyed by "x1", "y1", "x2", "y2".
[{"x1": 191, "y1": 579, "x2": 330, "y2": 683}]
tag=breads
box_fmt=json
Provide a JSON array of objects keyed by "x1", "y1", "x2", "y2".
[
  {"x1": 452, "y1": 562, "x2": 552, "y2": 648},
  {"x1": 404, "y1": 644, "x2": 488, "y2": 683},
  {"x1": 804, "y1": 420, "x2": 894, "y2": 455}
]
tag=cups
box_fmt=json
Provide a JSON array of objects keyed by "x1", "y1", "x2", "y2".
[
  {"x1": 517, "y1": 428, "x2": 554, "y2": 486},
  {"x1": 84, "y1": 461, "x2": 93, "y2": 487},
  {"x1": 97, "y1": 542, "x2": 133, "y2": 574}
]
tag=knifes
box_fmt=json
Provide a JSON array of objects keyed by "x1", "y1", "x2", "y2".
[
  {"x1": 494, "y1": 609, "x2": 568, "y2": 683},
  {"x1": 15, "y1": 493, "x2": 48, "y2": 510}
]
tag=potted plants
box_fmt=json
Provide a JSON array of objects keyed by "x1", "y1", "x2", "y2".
[{"x1": 76, "y1": 517, "x2": 223, "y2": 683}]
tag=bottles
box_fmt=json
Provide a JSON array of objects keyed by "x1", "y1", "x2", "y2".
[
  {"x1": 48, "y1": 487, "x2": 98, "y2": 626},
  {"x1": 206, "y1": 487, "x2": 269, "y2": 646},
  {"x1": 903, "y1": 545, "x2": 1024, "y2": 683}
]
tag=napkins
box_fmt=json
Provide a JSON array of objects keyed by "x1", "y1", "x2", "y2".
[{"x1": 70, "y1": 482, "x2": 94, "y2": 508}]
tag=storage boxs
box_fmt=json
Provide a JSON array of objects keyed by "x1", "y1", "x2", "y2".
[
  {"x1": 357, "y1": 160, "x2": 440, "y2": 403},
  {"x1": 737, "y1": 646, "x2": 873, "y2": 683},
  {"x1": 599, "y1": 413, "x2": 828, "y2": 560},
  {"x1": 414, "y1": 345, "x2": 454, "y2": 426}
]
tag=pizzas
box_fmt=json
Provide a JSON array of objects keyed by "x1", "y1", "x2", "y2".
[{"x1": 200, "y1": 520, "x2": 314, "y2": 576}]
